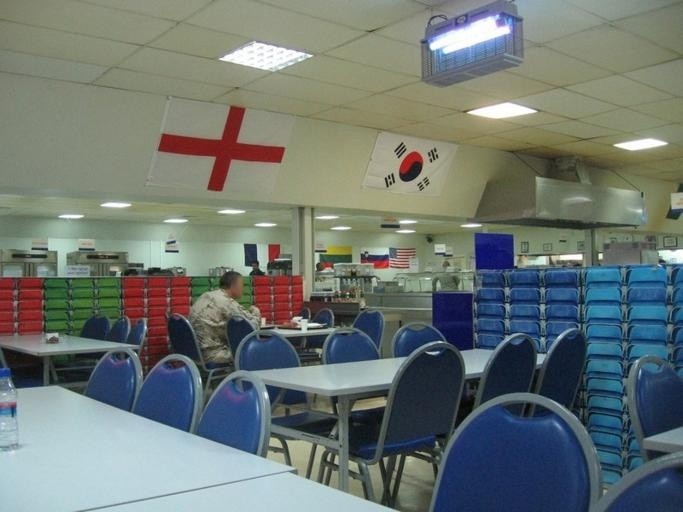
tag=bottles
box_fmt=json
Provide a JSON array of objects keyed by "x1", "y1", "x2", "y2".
[
  {"x1": 1, "y1": 367, "x2": 19, "y2": 451},
  {"x1": 330, "y1": 268, "x2": 362, "y2": 304}
]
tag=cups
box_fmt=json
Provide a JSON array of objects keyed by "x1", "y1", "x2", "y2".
[{"x1": 300, "y1": 319, "x2": 308, "y2": 332}]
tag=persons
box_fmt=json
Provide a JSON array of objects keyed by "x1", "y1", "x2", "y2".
[
  {"x1": 247, "y1": 260, "x2": 265, "y2": 276},
  {"x1": 185, "y1": 271, "x2": 263, "y2": 365},
  {"x1": 432, "y1": 260, "x2": 462, "y2": 292}
]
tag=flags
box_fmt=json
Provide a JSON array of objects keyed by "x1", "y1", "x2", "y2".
[
  {"x1": 313, "y1": 244, "x2": 353, "y2": 270},
  {"x1": 242, "y1": 242, "x2": 282, "y2": 268},
  {"x1": 360, "y1": 246, "x2": 389, "y2": 269},
  {"x1": 143, "y1": 92, "x2": 298, "y2": 207},
  {"x1": 389, "y1": 247, "x2": 417, "y2": 270},
  {"x1": 362, "y1": 130, "x2": 463, "y2": 203}
]
